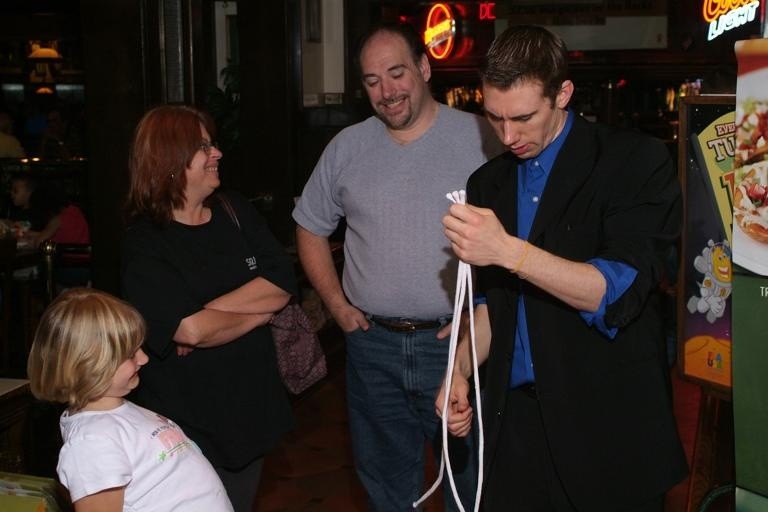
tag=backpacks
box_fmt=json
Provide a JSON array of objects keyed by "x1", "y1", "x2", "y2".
[{"x1": 269, "y1": 303, "x2": 329, "y2": 399}]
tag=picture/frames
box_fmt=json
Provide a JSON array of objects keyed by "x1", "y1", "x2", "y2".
[{"x1": 677, "y1": 95, "x2": 732, "y2": 401}]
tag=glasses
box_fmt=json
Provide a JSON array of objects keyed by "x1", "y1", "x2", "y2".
[{"x1": 200, "y1": 141, "x2": 216, "y2": 155}]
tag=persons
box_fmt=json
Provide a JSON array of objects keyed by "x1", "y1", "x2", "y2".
[
  {"x1": 7, "y1": 174, "x2": 46, "y2": 233},
  {"x1": 433, "y1": 22, "x2": 691, "y2": 510},
  {"x1": 30, "y1": 168, "x2": 95, "y2": 290},
  {"x1": 25, "y1": 285, "x2": 234, "y2": 512},
  {"x1": 290, "y1": 22, "x2": 508, "y2": 511},
  {"x1": 118, "y1": 102, "x2": 309, "y2": 512}
]
